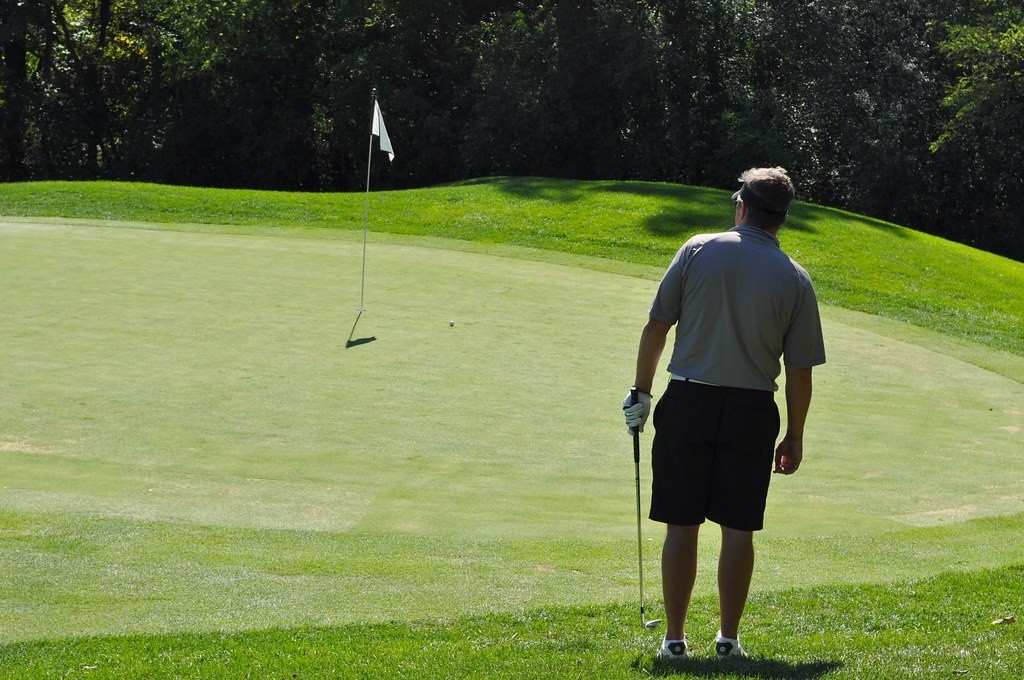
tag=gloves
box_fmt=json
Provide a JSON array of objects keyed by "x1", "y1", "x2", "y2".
[{"x1": 623, "y1": 389, "x2": 652, "y2": 435}]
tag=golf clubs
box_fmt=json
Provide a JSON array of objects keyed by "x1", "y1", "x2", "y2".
[{"x1": 629, "y1": 386, "x2": 663, "y2": 631}]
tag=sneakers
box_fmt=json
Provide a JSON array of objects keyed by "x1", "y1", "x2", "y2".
[
  {"x1": 657, "y1": 640, "x2": 689, "y2": 661},
  {"x1": 714, "y1": 641, "x2": 746, "y2": 657}
]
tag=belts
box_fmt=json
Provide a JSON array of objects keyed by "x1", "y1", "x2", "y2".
[{"x1": 669, "y1": 372, "x2": 720, "y2": 387}]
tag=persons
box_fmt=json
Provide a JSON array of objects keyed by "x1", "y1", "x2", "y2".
[{"x1": 624, "y1": 165, "x2": 827, "y2": 664}]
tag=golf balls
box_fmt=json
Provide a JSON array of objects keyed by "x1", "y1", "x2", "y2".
[{"x1": 450, "y1": 321, "x2": 454, "y2": 326}]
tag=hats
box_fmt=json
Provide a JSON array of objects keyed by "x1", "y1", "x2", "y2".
[{"x1": 732, "y1": 182, "x2": 790, "y2": 211}]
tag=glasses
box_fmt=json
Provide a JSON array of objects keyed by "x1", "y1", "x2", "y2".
[{"x1": 733, "y1": 200, "x2": 739, "y2": 206}]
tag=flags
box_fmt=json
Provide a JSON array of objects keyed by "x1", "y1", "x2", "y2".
[{"x1": 371, "y1": 99, "x2": 395, "y2": 162}]
